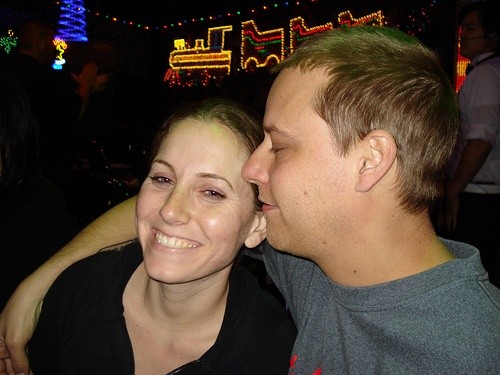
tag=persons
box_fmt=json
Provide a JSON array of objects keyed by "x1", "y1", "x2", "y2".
[
  {"x1": 436, "y1": 0, "x2": 500, "y2": 291},
  {"x1": 28, "y1": 100, "x2": 297, "y2": 375},
  {"x1": 0, "y1": 24, "x2": 500, "y2": 375},
  {"x1": 0, "y1": 20, "x2": 107, "y2": 276},
  {"x1": 72, "y1": 22, "x2": 133, "y2": 166}
]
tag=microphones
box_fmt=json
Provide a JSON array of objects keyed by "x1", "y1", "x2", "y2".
[{"x1": 454, "y1": 36, "x2": 484, "y2": 42}]
